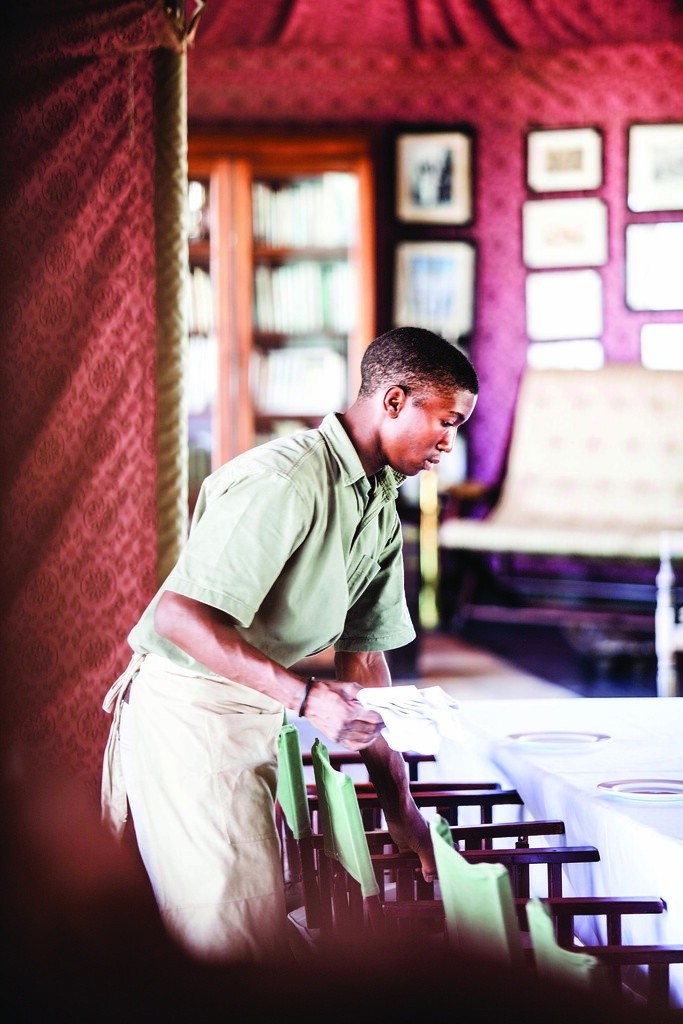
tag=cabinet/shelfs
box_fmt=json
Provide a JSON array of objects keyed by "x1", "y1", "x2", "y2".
[{"x1": 182, "y1": 136, "x2": 377, "y2": 521}]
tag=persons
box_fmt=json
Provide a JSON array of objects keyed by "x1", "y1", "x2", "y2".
[{"x1": 99, "y1": 325, "x2": 480, "y2": 958}]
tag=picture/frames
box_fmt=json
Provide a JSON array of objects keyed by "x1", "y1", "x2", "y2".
[{"x1": 378, "y1": 118, "x2": 683, "y2": 512}]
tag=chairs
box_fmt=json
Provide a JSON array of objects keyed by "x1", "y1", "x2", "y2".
[{"x1": 272, "y1": 708, "x2": 683, "y2": 1022}]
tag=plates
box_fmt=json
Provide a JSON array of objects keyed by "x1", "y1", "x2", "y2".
[
  {"x1": 596, "y1": 778, "x2": 683, "y2": 805},
  {"x1": 507, "y1": 731, "x2": 612, "y2": 754}
]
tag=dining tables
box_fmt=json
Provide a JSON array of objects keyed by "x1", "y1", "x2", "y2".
[{"x1": 468, "y1": 696, "x2": 683, "y2": 995}]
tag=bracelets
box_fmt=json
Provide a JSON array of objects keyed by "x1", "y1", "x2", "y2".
[{"x1": 299, "y1": 676, "x2": 315, "y2": 717}]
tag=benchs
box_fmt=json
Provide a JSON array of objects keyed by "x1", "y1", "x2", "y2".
[{"x1": 438, "y1": 369, "x2": 683, "y2": 644}]
